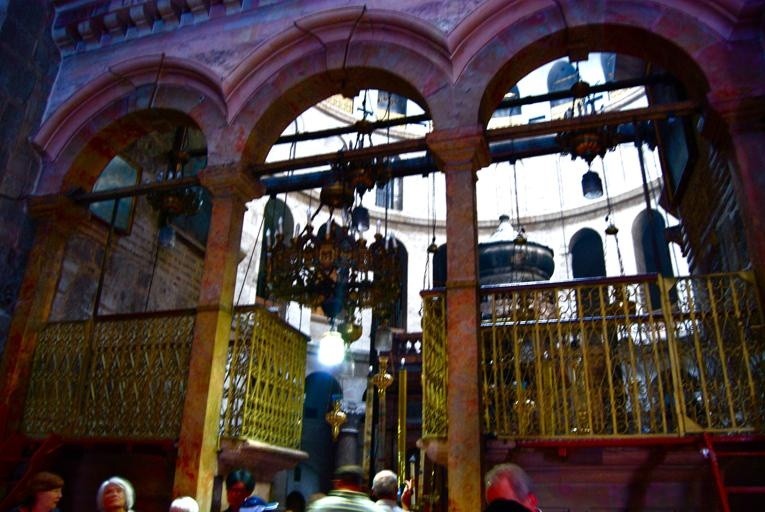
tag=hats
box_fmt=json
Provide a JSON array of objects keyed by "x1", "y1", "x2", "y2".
[
  {"x1": 335, "y1": 464, "x2": 365, "y2": 478},
  {"x1": 238, "y1": 495, "x2": 279, "y2": 512}
]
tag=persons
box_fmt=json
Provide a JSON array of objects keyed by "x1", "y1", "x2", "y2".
[{"x1": 0, "y1": 463, "x2": 543, "y2": 512}]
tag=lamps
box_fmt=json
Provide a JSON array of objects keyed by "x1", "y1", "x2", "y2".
[
  {"x1": 554, "y1": 39, "x2": 622, "y2": 201},
  {"x1": 143, "y1": 123, "x2": 204, "y2": 228},
  {"x1": 260, "y1": 89, "x2": 405, "y2": 328}
]
